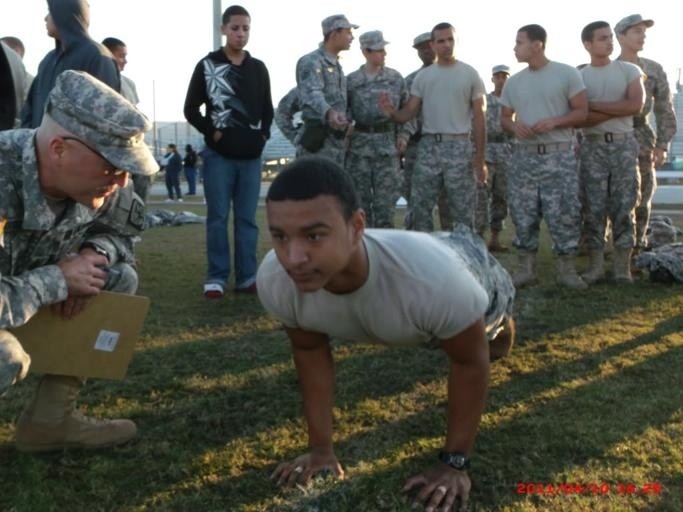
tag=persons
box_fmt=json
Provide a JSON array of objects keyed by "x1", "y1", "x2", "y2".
[
  {"x1": 0, "y1": 70, "x2": 160, "y2": 452},
  {"x1": 0, "y1": 2, "x2": 682, "y2": 297},
  {"x1": 255, "y1": 157, "x2": 514, "y2": 511}
]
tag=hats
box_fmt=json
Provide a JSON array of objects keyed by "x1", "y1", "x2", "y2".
[
  {"x1": 492, "y1": 65, "x2": 510, "y2": 77},
  {"x1": 43, "y1": 70, "x2": 161, "y2": 176},
  {"x1": 614, "y1": 13, "x2": 654, "y2": 35},
  {"x1": 321, "y1": 14, "x2": 359, "y2": 36},
  {"x1": 358, "y1": 31, "x2": 388, "y2": 50},
  {"x1": 412, "y1": 33, "x2": 431, "y2": 48}
]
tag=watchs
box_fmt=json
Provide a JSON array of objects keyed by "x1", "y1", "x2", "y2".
[
  {"x1": 439, "y1": 451, "x2": 470, "y2": 471},
  {"x1": 79, "y1": 242, "x2": 108, "y2": 260}
]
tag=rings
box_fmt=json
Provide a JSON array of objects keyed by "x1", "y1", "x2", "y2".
[{"x1": 293, "y1": 465, "x2": 302, "y2": 474}]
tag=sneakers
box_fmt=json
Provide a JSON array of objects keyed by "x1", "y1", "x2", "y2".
[
  {"x1": 203, "y1": 283, "x2": 224, "y2": 298},
  {"x1": 489, "y1": 318, "x2": 515, "y2": 361}
]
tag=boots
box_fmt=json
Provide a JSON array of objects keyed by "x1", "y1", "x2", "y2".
[
  {"x1": 554, "y1": 248, "x2": 643, "y2": 290},
  {"x1": 489, "y1": 234, "x2": 508, "y2": 252},
  {"x1": 513, "y1": 248, "x2": 539, "y2": 286},
  {"x1": 13, "y1": 375, "x2": 138, "y2": 456}
]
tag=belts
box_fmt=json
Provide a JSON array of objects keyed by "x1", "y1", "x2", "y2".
[
  {"x1": 634, "y1": 117, "x2": 644, "y2": 127},
  {"x1": 487, "y1": 135, "x2": 510, "y2": 142},
  {"x1": 528, "y1": 142, "x2": 570, "y2": 153},
  {"x1": 352, "y1": 124, "x2": 395, "y2": 131},
  {"x1": 424, "y1": 133, "x2": 467, "y2": 141},
  {"x1": 586, "y1": 132, "x2": 626, "y2": 142}
]
